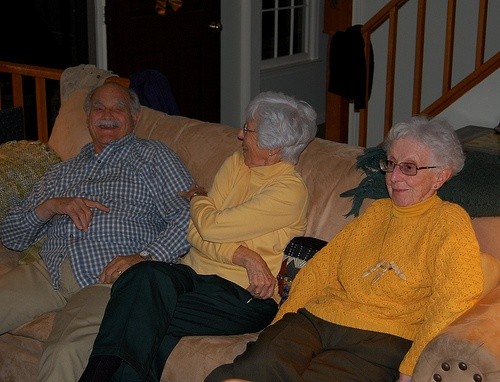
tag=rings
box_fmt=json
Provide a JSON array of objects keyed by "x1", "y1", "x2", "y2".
[
  {"x1": 253, "y1": 290, "x2": 262, "y2": 295},
  {"x1": 114, "y1": 270, "x2": 122, "y2": 275},
  {"x1": 191, "y1": 187, "x2": 195, "y2": 191}
]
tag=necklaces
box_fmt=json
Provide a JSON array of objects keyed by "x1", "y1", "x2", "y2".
[{"x1": 360, "y1": 214, "x2": 421, "y2": 286}]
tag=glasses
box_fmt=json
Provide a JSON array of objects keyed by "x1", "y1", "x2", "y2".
[
  {"x1": 243, "y1": 122, "x2": 256, "y2": 133},
  {"x1": 379, "y1": 159, "x2": 437, "y2": 176}
]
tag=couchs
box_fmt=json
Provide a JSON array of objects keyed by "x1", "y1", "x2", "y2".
[{"x1": 0, "y1": 65, "x2": 500, "y2": 382}]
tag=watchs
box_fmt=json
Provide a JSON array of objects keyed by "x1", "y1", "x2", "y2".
[
  {"x1": 137, "y1": 250, "x2": 152, "y2": 260},
  {"x1": 190, "y1": 190, "x2": 207, "y2": 201}
]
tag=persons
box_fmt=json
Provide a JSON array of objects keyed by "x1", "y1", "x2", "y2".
[
  {"x1": 204, "y1": 116, "x2": 484, "y2": 382},
  {"x1": 78, "y1": 91, "x2": 318, "y2": 382},
  {"x1": 0, "y1": 83, "x2": 201, "y2": 382}
]
